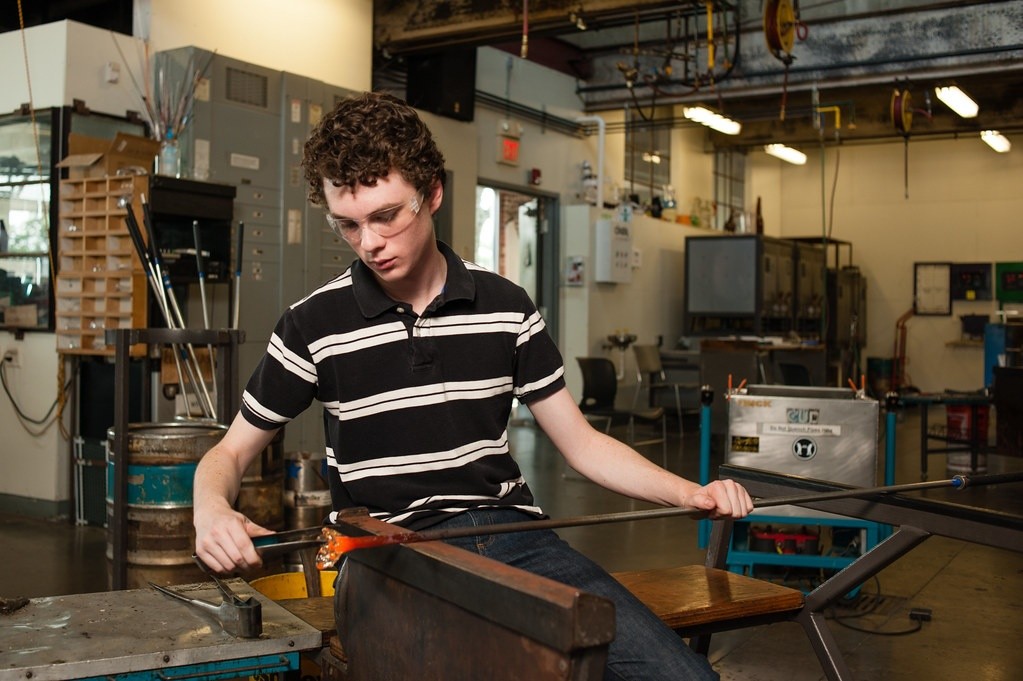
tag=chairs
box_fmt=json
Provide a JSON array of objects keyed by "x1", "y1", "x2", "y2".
[
  {"x1": 575, "y1": 356, "x2": 668, "y2": 471},
  {"x1": 628, "y1": 343, "x2": 700, "y2": 442}
]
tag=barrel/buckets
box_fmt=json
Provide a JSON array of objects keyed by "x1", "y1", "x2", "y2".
[
  {"x1": 106, "y1": 420, "x2": 331, "y2": 590},
  {"x1": 248, "y1": 571, "x2": 338, "y2": 600},
  {"x1": 866, "y1": 356, "x2": 903, "y2": 422},
  {"x1": 946, "y1": 393, "x2": 989, "y2": 472}
]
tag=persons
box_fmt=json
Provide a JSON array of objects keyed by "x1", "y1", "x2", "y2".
[{"x1": 190, "y1": 93, "x2": 752, "y2": 681}]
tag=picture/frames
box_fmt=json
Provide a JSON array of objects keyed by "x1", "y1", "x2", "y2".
[{"x1": 913, "y1": 261, "x2": 953, "y2": 316}]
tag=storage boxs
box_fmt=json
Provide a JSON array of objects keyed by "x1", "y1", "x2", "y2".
[
  {"x1": 149, "y1": 175, "x2": 239, "y2": 218},
  {"x1": 54, "y1": 130, "x2": 163, "y2": 178},
  {"x1": 148, "y1": 214, "x2": 234, "y2": 287}
]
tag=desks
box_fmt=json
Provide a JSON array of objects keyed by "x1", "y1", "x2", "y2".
[
  {"x1": 882, "y1": 390, "x2": 997, "y2": 482},
  {"x1": 0, "y1": 577, "x2": 324, "y2": 681},
  {"x1": 276, "y1": 565, "x2": 805, "y2": 681}
]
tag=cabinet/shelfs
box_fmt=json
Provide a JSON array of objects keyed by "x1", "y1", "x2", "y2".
[
  {"x1": 727, "y1": 505, "x2": 880, "y2": 602},
  {"x1": 54, "y1": 178, "x2": 149, "y2": 357}
]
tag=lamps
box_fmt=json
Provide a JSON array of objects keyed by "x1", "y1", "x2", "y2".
[
  {"x1": 980, "y1": 128, "x2": 1013, "y2": 155},
  {"x1": 934, "y1": 77, "x2": 980, "y2": 119},
  {"x1": 763, "y1": 143, "x2": 807, "y2": 166},
  {"x1": 684, "y1": 103, "x2": 742, "y2": 135}
]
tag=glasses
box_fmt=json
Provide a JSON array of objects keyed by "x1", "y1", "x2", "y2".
[{"x1": 326, "y1": 187, "x2": 425, "y2": 244}]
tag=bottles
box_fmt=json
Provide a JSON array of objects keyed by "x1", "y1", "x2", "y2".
[
  {"x1": 157, "y1": 132, "x2": 181, "y2": 178},
  {"x1": 753, "y1": 197, "x2": 764, "y2": 238},
  {"x1": 723, "y1": 210, "x2": 736, "y2": 231},
  {"x1": 691, "y1": 197, "x2": 716, "y2": 228}
]
